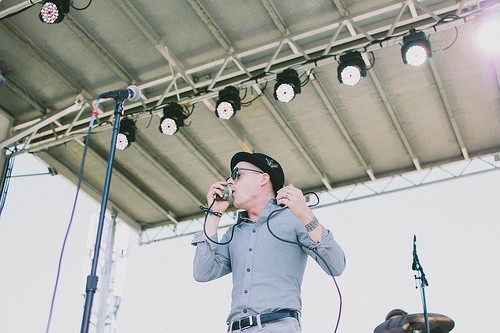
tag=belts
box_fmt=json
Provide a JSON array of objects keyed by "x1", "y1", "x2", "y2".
[{"x1": 228, "y1": 310, "x2": 298, "y2": 331}]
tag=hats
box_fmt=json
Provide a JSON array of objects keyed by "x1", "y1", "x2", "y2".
[{"x1": 231, "y1": 152, "x2": 285, "y2": 194}]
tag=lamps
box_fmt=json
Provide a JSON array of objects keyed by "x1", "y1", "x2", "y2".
[
  {"x1": 215, "y1": 86, "x2": 241, "y2": 120},
  {"x1": 116, "y1": 118, "x2": 136, "y2": 150},
  {"x1": 401, "y1": 28, "x2": 432, "y2": 67},
  {"x1": 337, "y1": 51, "x2": 367, "y2": 86},
  {"x1": 159, "y1": 102, "x2": 187, "y2": 136},
  {"x1": 274, "y1": 68, "x2": 302, "y2": 103},
  {"x1": 39, "y1": 0, "x2": 70, "y2": 24}
]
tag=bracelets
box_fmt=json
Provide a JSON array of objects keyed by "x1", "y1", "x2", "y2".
[
  {"x1": 305, "y1": 217, "x2": 319, "y2": 232},
  {"x1": 213, "y1": 212, "x2": 222, "y2": 218}
]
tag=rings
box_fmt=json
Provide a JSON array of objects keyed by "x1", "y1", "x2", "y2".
[{"x1": 285, "y1": 194, "x2": 288, "y2": 199}]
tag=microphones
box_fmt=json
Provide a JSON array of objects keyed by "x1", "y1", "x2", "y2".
[
  {"x1": 99, "y1": 85, "x2": 141, "y2": 102},
  {"x1": 216, "y1": 185, "x2": 232, "y2": 201}
]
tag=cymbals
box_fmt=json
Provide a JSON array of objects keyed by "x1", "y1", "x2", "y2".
[{"x1": 373, "y1": 313, "x2": 455, "y2": 333}]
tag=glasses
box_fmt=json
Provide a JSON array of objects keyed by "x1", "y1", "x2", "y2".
[{"x1": 226, "y1": 167, "x2": 264, "y2": 180}]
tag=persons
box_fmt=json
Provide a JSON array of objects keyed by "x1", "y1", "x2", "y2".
[{"x1": 191, "y1": 152, "x2": 346, "y2": 333}]
